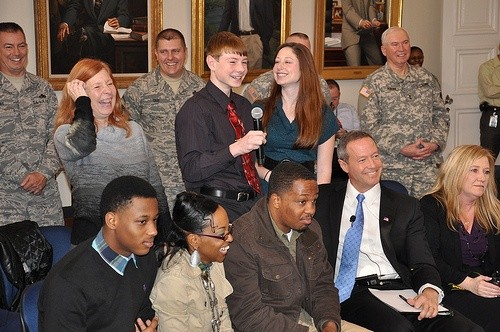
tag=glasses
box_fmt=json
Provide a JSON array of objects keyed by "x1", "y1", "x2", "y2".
[{"x1": 196, "y1": 223, "x2": 235, "y2": 241}]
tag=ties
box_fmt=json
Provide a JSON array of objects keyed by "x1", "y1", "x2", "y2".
[
  {"x1": 226, "y1": 100, "x2": 261, "y2": 193},
  {"x1": 334, "y1": 194, "x2": 365, "y2": 303}
]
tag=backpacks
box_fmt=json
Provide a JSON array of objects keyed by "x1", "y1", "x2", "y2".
[{"x1": 0, "y1": 220, "x2": 53, "y2": 312}]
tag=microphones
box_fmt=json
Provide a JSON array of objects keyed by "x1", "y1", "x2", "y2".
[
  {"x1": 250, "y1": 107, "x2": 265, "y2": 165},
  {"x1": 350, "y1": 215, "x2": 356, "y2": 222}
]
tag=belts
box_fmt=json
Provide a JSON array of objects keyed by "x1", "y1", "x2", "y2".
[
  {"x1": 200, "y1": 187, "x2": 257, "y2": 201},
  {"x1": 240, "y1": 30, "x2": 257, "y2": 35}
]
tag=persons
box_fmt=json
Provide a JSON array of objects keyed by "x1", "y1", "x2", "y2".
[
  {"x1": 0, "y1": 22, "x2": 65, "y2": 227},
  {"x1": 359, "y1": 27, "x2": 449, "y2": 199},
  {"x1": 222, "y1": 163, "x2": 374, "y2": 332},
  {"x1": 312, "y1": 131, "x2": 487, "y2": 332},
  {"x1": 419, "y1": 145, "x2": 500, "y2": 332},
  {"x1": 175, "y1": 32, "x2": 271, "y2": 226},
  {"x1": 37, "y1": 176, "x2": 163, "y2": 332},
  {"x1": 121, "y1": 29, "x2": 206, "y2": 219},
  {"x1": 478, "y1": 54, "x2": 500, "y2": 164},
  {"x1": 149, "y1": 191, "x2": 235, "y2": 332},
  {"x1": 54, "y1": 59, "x2": 172, "y2": 246},
  {"x1": 243, "y1": 33, "x2": 340, "y2": 198},
  {"x1": 326, "y1": 79, "x2": 361, "y2": 169},
  {"x1": 408, "y1": 47, "x2": 423, "y2": 67}
]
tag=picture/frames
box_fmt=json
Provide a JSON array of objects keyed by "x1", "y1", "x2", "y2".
[
  {"x1": 34, "y1": 0, "x2": 163, "y2": 89},
  {"x1": 191, "y1": 0, "x2": 291, "y2": 83},
  {"x1": 331, "y1": 1, "x2": 338, "y2": 20},
  {"x1": 314, "y1": 0, "x2": 403, "y2": 80},
  {"x1": 334, "y1": 7, "x2": 343, "y2": 19}
]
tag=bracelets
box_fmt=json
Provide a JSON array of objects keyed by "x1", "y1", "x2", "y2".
[{"x1": 264, "y1": 171, "x2": 270, "y2": 181}]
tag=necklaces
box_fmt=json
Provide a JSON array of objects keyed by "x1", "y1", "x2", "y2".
[
  {"x1": 463, "y1": 233, "x2": 488, "y2": 260},
  {"x1": 201, "y1": 270, "x2": 222, "y2": 332}
]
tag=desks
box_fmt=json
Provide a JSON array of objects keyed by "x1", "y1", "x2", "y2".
[{"x1": 109, "y1": 33, "x2": 148, "y2": 73}]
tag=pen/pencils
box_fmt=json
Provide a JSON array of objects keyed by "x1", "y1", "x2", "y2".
[{"x1": 399, "y1": 295, "x2": 414, "y2": 307}]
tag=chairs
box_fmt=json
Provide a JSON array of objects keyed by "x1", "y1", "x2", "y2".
[{"x1": 0, "y1": 226, "x2": 78, "y2": 332}]
